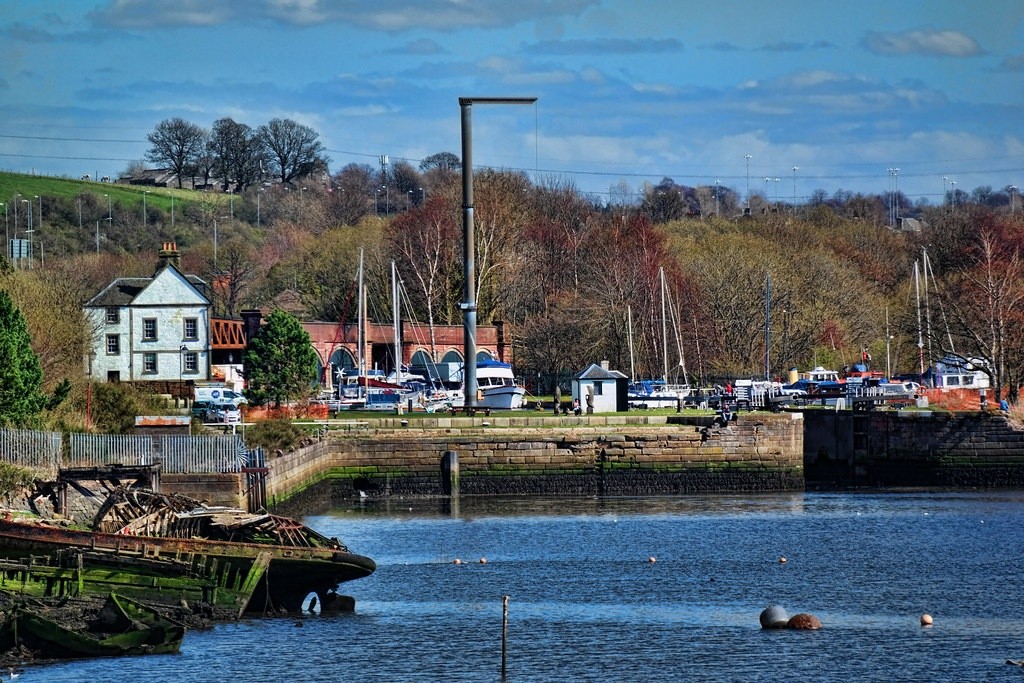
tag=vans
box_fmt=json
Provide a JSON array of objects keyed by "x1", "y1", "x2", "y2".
[{"x1": 192, "y1": 385, "x2": 248, "y2": 423}]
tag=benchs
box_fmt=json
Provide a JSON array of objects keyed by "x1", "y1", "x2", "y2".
[
  {"x1": 328, "y1": 409, "x2": 338, "y2": 418},
  {"x1": 566, "y1": 409, "x2": 581, "y2": 416},
  {"x1": 452, "y1": 406, "x2": 491, "y2": 416}
]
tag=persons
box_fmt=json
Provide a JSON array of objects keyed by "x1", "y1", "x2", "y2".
[
  {"x1": 1000, "y1": 398, "x2": 1009, "y2": 413},
  {"x1": 772, "y1": 375, "x2": 781, "y2": 382},
  {"x1": 722, "y1": 405, "x2": 732, "y2": 420},
  {"x1": 726, "y1": 382, "x2": 732, "y2": 394},
  {"x1": 223, "y1": 410, "x2": 229, "y2": 435},
  {"x1": 573, "y1": 398, "x2": 581, "y2": 415}
]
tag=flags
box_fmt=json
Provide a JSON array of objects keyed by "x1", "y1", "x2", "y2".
[{"x1": 863, "y1": 352, "x2": 871, "y2": 360}]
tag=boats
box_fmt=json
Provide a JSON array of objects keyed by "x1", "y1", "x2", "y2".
[{"x1": 0, "y1": 482, "x2": 375, "y2": 613}]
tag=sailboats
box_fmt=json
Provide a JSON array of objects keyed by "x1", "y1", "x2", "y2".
[
  {"x1": 751, "y1": 244, "x2": 997, "y2": 396},
  {"x1": 318, "y1": 248, "x2": 525, "y2": 409},
  {"x1": 626, "y1": 264, "x2": 704, "y2": 408}
]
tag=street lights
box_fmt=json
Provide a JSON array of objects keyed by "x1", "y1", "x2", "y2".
[
  {"x1": 97, "y1": 194, "x2": 113, "y2": 254},
  {"x1": 0, "y1": 194, "x2": 44, "y2": 271},
  {"x1": 715, "y1": 154, "x2": 799, "y2": 218},
  {"x1": 375, "y1": 186, "x2": 426, "y2": 214},
  {"x1": 1010, "y1": 185, "x2": 1018, "y2": 203},
  {"x1": 144, "y1": 190, "x2": 151, "y2": 227},
  {"x1": 257, "y1": 188, "x2": 264, "y2": 227},
  {"x1": 942, "y1": 177, "x2": 957, "y2": 203},
  {"x1": 168, "y1": 191, "x2": 174, "y2": 225},
  {"x1": 886, "y1": 168, "x2": 900, "y2": 225},
  {"x1": 226, "y1": 189, "x2": 233, "y2": 221}
]
